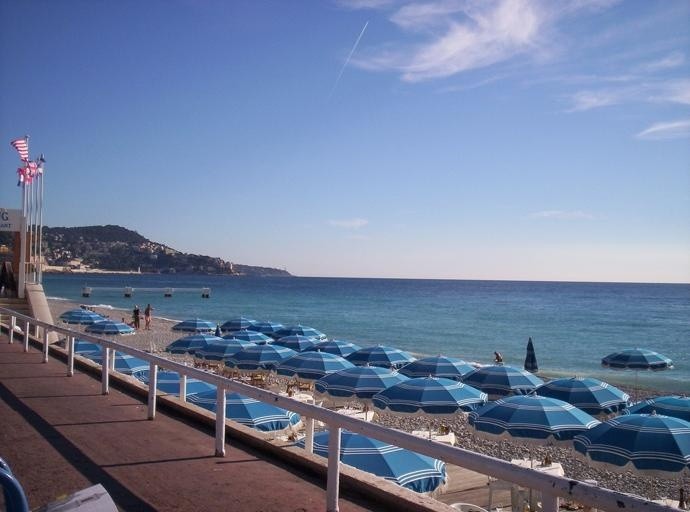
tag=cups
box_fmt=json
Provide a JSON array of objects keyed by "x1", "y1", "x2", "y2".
[{"x1": 496, "y1": 504, "x2": 504, "y2": 512}]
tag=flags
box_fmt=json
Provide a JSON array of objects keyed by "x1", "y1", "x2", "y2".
[{"x1": 10, "y1": 138, "x2": 44, "y2": 187}]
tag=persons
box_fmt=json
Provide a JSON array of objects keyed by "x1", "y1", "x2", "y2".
[
  {"x1": 145, "y1": 303, "x2": 153, "y2": 330},
  {"x1": 132, "y1": 305, "x2": 140, "y2": 329}
]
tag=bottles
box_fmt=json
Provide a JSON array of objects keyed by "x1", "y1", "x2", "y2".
[
  {"x1": 438, "y1": 421, "x2": 450, "y2": 435},
  {"x1": 678, "y1": 488, "x2": 690, "y2": 511},
  {"x1": 542, "y1": 450, "x2": 552, "y2": 468}
]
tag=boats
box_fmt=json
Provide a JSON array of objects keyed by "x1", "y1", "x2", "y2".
[
  {"x1": 124, "y1": 286, "x2": 135, "y2": 297},
  {"x1": 200, "y1": 287, "x2": 211, "y2": 299},
  {"x1": 164, "y1": 287, "x2": 175, "y2": 297},
  {"x1": 79, "y1": 286, "x2": 92, "y2": 298}
]
